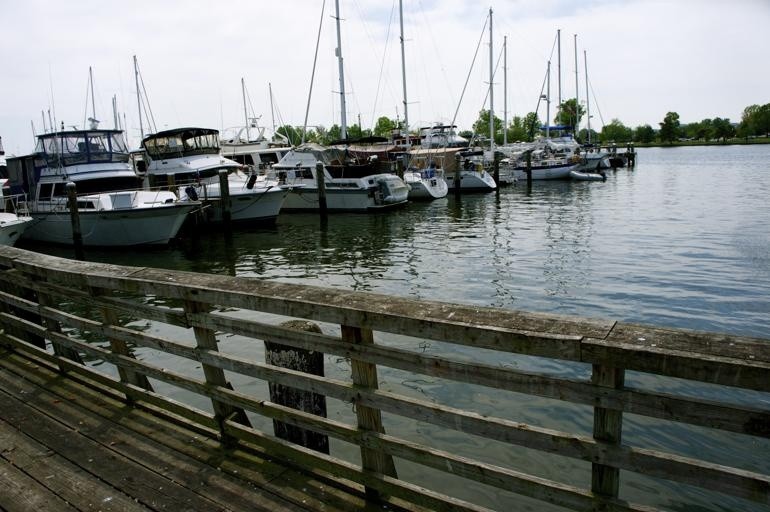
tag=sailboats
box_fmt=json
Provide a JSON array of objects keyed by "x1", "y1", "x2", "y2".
[{"x1": 29, "y1": 0, "x2": 612, "y2": 210}]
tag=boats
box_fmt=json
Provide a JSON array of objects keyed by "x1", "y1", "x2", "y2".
[
  {"x1": 0, "y1": 129, "x2": 208, "y2": 247},
  {"x1": 0, "y1": 192, "x2": 34, "y2": 248},
  {"x1": 138, "y1": 128, "x2": 308, "y2": 227}
]
